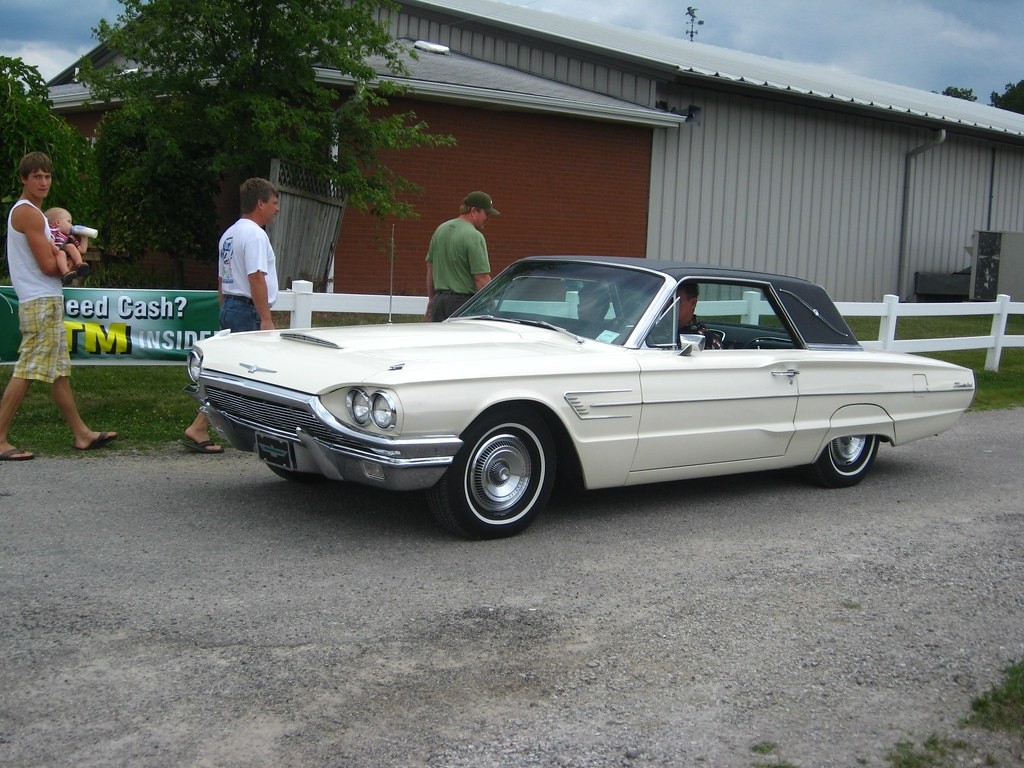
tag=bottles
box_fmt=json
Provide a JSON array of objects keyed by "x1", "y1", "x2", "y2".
[{"x1": 70, "y1": 225, "x2": 98, "y2": 238}]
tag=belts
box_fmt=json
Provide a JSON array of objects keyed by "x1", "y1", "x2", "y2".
[
  {"x1": 437, "y1": 291, "x2": 461, "y2": 294},
  {"x1": 225, "y1": 295, "x2": 254, "y2": 305}
]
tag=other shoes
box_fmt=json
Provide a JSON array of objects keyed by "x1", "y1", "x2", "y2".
[
  {"x1": 71, "y1": 262, "x2": 90, "y2": 275},
  {"x1": 63, "y1": 270, "x2": 77, "y2": 286}
]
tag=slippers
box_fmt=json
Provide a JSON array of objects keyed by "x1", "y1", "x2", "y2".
[
  {"x1": 0, "y1": 448, "x2": 35, "y2": 461},
  {"x1": 70, "y1": 431, "x2": 118, "y2": 450},
  {"x1": 177, "y1": 439, "x2": 225, "y2": 453}
]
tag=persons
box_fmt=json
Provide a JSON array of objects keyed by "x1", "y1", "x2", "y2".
[
  {"x1": 576, "y1": 283, "x2": 610, "y2": 324},
  {"x1": 0, "y1": 151, "x2": 119, "y2": 462},
  {"x1": 425, "y1": 191, "x2": 503, "y2": 322},
  {"x1": 44, "y1": 208, "x2": 90, "y2": 285},
  {"x1": 177, "y1": 178, "x2": 279, "y2": 452},
  {"x1": 631, "y1": 281, "x2": 722, "y2": 351}
]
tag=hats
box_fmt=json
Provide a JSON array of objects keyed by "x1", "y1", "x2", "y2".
[{"x1": 464, "y1": 190, "x2": 500, "y2": 216}]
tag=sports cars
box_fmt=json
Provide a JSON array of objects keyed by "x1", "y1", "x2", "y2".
[{"x1": 183, "y1": 253, "x2": 977, "y2": 541}]
